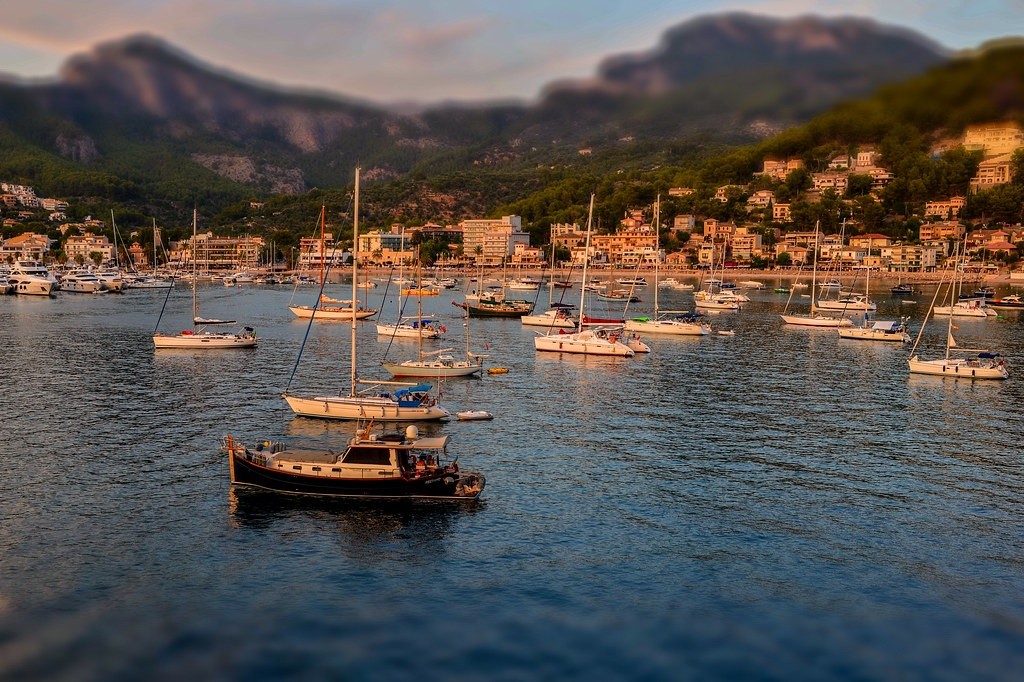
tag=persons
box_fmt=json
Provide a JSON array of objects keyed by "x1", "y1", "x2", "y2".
[{"x1": 415, "y1": 453, "x2": 427, "y2": 478}]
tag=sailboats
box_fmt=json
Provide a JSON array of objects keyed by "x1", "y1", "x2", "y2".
[
  {"x1": 109, "y1": 206, "x2": 182, "y2": 287},
  {"x1": 620, "y1": 202, "x2": 713, "y2": 336},
  {"x1": 151, "y1": 209, "x2": 260, "y2": 349},
  {"x1": 534, "y1": 194, "x2": 652, "y2": 355},
  {"x1": 288, "y1": 205, "x2": 378, "y2": 320},
  {"x1": 836, "y1": 239, "x2": 911, "y2": 345},
  {"x1": 780, "y1": 221, "x2": 854, "y2": 328},
  {"x1": 377, "y1": 229, "x2": 447, "y2": 340},
  {"x1": 282, "y1": 166, "x2": 450, "y2": 422},
  {"x1": 451, "y1": 230, "x2": 547, "y2": 318},
  {"x1": 381, "y1": 246, "x2": 489, "y2": 377},
  {"x1": 907, "y1": 233, "x2": 1024, "y2": 380},
  {"x1": 519, "y1": 220, "x2": 581, "y2": 327},
  {"x1": 889, "y1": 244, "x2": 913, "y2": 295},
  {"x1": 817, "y1": 218, "x2": 878, "y2": 310},
  {"x1": 694, "y1": 226, "x2": 740, "y2": 309}
]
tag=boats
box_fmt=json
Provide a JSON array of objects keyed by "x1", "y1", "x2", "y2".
[
  {"x1": 456, "y1": 410, "x2": 494, "y2": 421},
  {"x1": 791, "y1": 283, "x2": 809, "y2": 289},
  {"x1": 703, "y1": 278, "x2": 790, "y2": 294},
  {"x1": 816, "y1": 278, "x2": 844, "y2": 289},
  {"x1": 0, "y1": 255, "x2": 212, "y2": 297},
  {"x1": 221, "y1": 271, "x2": 693, "y2": 303},
  {"x1": 218, "y1": 413, "x2": 485, "y2": 510}
]
